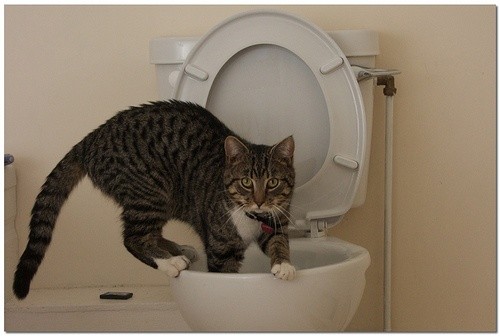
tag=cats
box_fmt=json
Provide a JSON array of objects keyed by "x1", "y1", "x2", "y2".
[{"x1": 11, "y1": 97, "x2": 298, "y2": 301}]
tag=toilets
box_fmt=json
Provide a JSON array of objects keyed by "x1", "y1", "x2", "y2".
[{"x1": 147, "y1": 7, "x2": 380, "y2": 332}]
